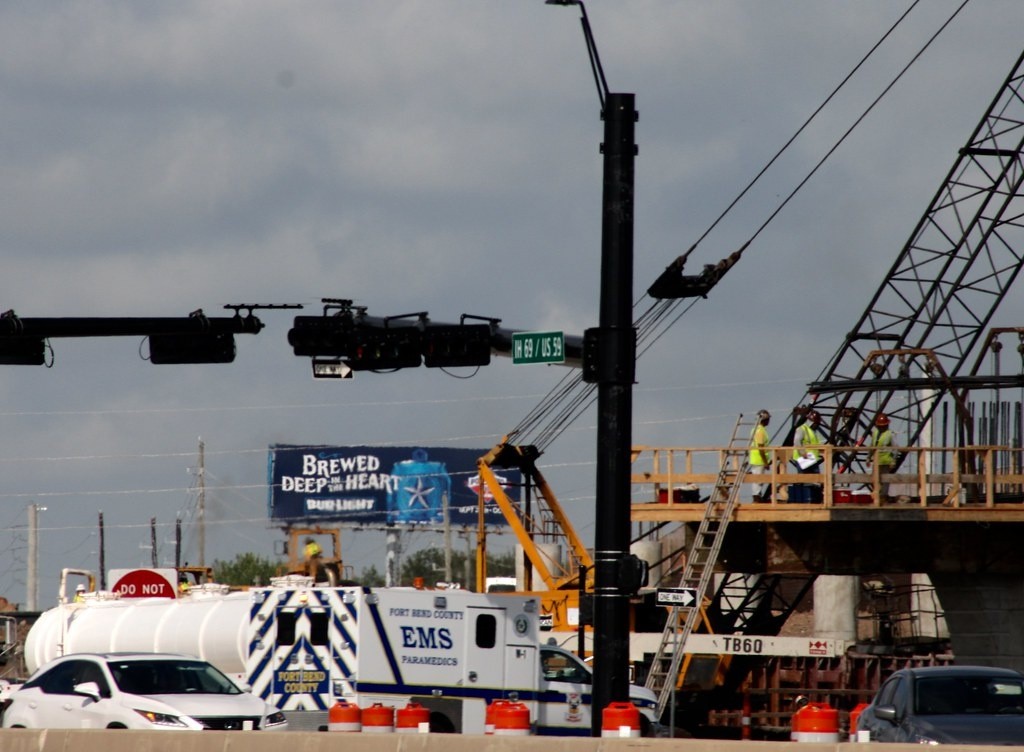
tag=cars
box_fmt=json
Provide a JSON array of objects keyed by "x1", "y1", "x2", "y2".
[{"x1": 855, "y1": 666, "x2": 1024, "y2": 744}]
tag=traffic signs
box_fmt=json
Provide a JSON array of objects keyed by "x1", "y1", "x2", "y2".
[
  {"x1": 312, "y1": 359, "x2": 353, "y2": 379},
  {"x1": 655, "y1": 588, "x2": 698, "y2": 608}
]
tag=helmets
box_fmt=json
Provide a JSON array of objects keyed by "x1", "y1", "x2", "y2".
[
  {"x1": 875, "y1": 414, "x2": 890, "y2": 426},
  {"x1": 756, "y1": 409, "x2": 771, "y2": 420},
  {"x1": 806, "y1": 410, "x2": 822, "y2": 423}
]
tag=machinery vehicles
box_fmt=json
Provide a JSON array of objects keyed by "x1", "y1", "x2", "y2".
[{"x1": 168, "y1": 1, "x2": 968, "y2": 675}]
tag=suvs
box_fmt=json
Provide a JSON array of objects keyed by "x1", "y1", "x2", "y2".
[{"x1": 3, "y1": 652, "x2": 288, "y2": 730}]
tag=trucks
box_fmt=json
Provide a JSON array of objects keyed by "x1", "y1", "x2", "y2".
[{"x1": 247, "y1": 576, "x2": 660, "y2": 737}]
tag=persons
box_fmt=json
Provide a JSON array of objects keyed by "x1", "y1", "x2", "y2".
[
  {"x1": 794, "y1": 411, "x2": 823, "y2": 504},
  {"x1": 866, "y1": 413, "x2": 899, "y2": 507},
  {"x1": 178, "y1": 573, "x2": 193, "y2": 594},
  {"x1": 750, "y1": 410, "x2": 772, "y2": 503},
  {"x1": 72, "y1": 584, "x2": 85, "y2": 603},
  {"x1": 303, "y1": 538, "x2": 323, "y2": 558}
]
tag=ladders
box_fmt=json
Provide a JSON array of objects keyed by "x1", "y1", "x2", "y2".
[{"x1": 645, "y1": 411, "x2": 762, "y2": 724}]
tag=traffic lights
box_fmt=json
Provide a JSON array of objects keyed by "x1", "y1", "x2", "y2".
[{"x1": 288, "y1": 296, "x2": 501, "y2": 371}]
